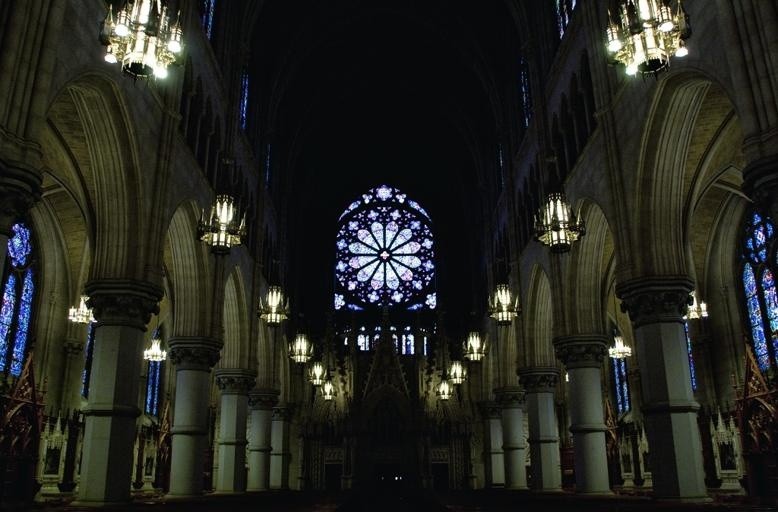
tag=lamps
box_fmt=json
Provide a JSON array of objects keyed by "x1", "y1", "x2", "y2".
[
  {"x1": 98, "y1": 0, "x2": 186, "y2": 83},
  {"x1": 602, "y1": 0, "x2": 690, "y2": 86},
  {"x1": 195, "y1": 177, "x2": 336, "y2": 403},
  {"x1": 435, "y1": 171, "x2": 586, "y2": 402}
]
